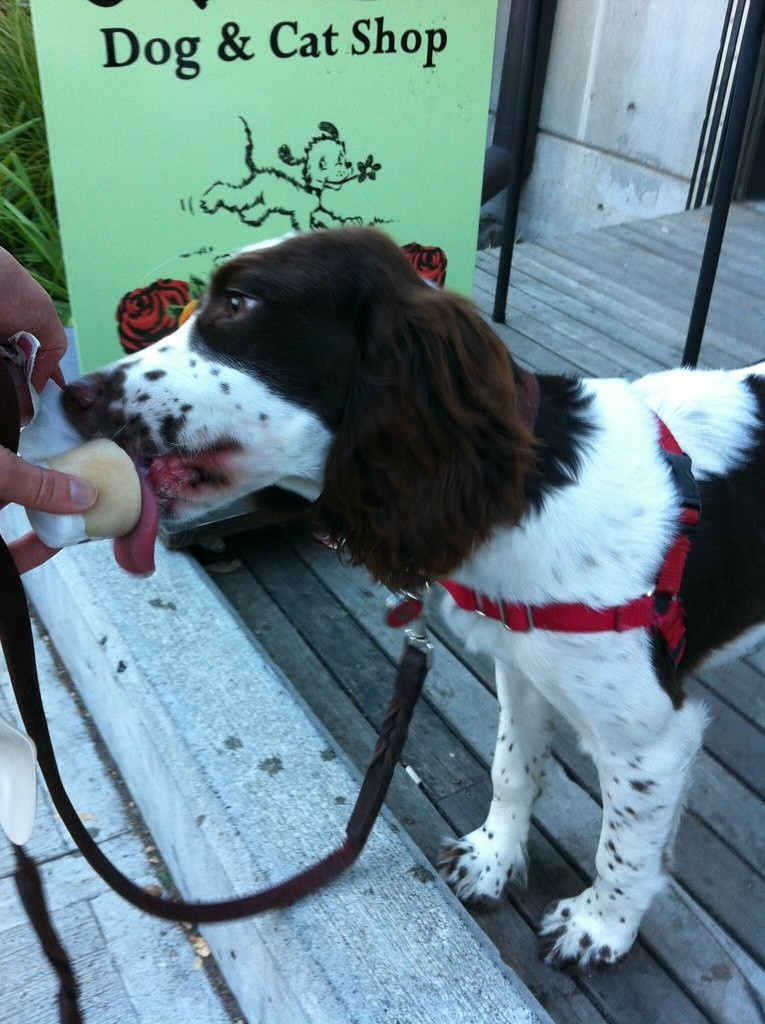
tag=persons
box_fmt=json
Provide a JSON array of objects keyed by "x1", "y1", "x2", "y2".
[{"x1": 1, "y1": 249, "x2": 100, "y2": 580}]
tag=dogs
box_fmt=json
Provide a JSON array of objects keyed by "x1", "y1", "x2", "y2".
[{"x1": 58, "y1": 228, "x2": 763, "y2": 974}]
